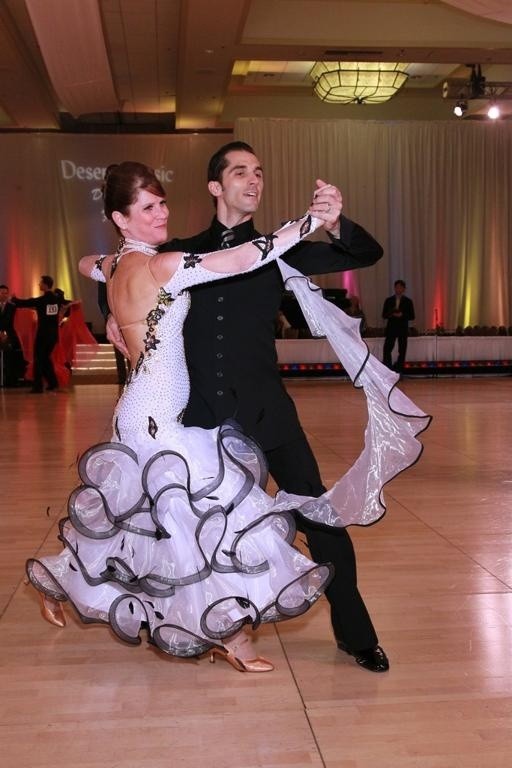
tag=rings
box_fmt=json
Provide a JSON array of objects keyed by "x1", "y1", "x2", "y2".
[{"x1": 325, "y1": 204, "x2": 333, "y2": 214}]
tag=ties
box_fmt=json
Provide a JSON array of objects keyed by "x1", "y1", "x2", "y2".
[{"x1": 220, "y1": 231, "x2": 234, "y2": 250}]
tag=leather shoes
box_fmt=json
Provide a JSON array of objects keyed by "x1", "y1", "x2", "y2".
[{"x1": 337, "y1": 641, "x2": 389, "y2": 672}]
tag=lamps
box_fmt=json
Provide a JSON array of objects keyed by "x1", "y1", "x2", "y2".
[
  {"x1": 307, "y1": 60, "x2": 409, "y2": 106},
  {"x1": 453, "y1": 93, "x2": 468, "y2": 117}
]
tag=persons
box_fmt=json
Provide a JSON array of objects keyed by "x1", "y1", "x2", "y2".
[
  {"x1": 342, "y1": 293, "x2": 367, "y2": 331},
  {"x1": 0, "y1": 285, "x2": 24, "y2": 388},
  {"x1": 379, "y1": 279, "x2": 416, "y2": 382},
  {"x1": 21, "y1": 154, "x2": 345, "y2": 674},
  {"x1": 11, "y1": 287, "x2": 87, "y2": 393},
  {"x1": 10, "y1": 273, "x2": 63, "y2": 395},
  {"x1": 95, "y1": 139, "x2": 392, "y2": 672}
]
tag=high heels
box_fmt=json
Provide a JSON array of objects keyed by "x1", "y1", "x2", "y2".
[
  {"x1": 23, "y1": 577, "x2": 66, "y2": 626},
  {"x1": 208, "y1": 630, "x2": 274, "y2": 672}
]
